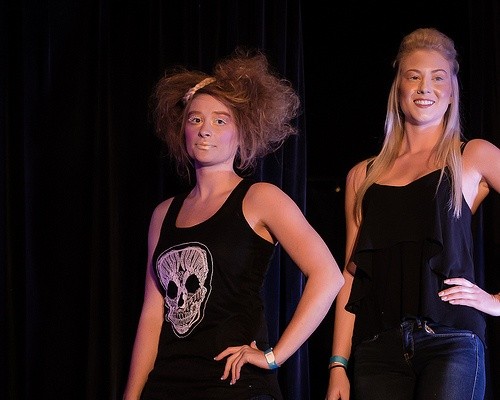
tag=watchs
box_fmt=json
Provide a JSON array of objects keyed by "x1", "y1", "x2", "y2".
[{"x1": 264, "y1": 347, "x2": 280, "y2": 369}]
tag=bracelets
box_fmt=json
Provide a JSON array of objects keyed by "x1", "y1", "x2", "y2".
[{"x1": 328, "y1": 356, "x2": 349, "y2": 371}]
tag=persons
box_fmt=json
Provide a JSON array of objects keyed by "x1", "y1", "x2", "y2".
[
  {"x1": 123, "y1": 53, "x2": 344, "y2": 400},
  {"x1": 326, "y1": 28, "x2": 499, "y2": 400}
]
